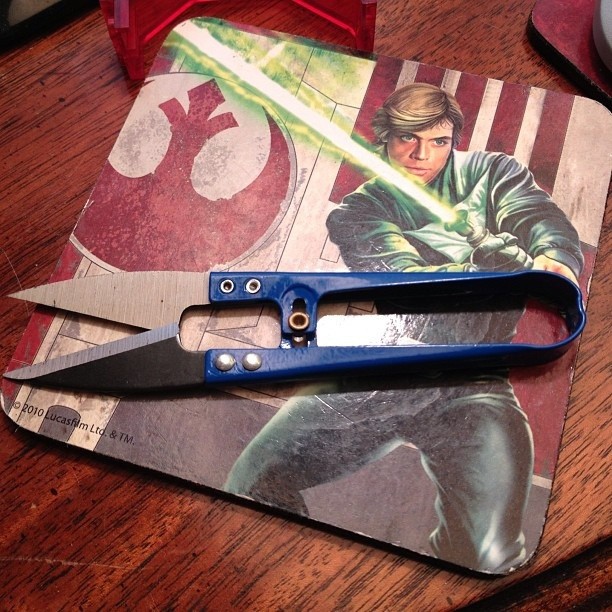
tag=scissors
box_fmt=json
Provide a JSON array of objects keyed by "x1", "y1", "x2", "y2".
[{"x1": 3, "y1": 271, "x2": 587, "y2": 396}]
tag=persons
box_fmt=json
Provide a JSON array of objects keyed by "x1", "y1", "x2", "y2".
[{"x1": 220, "y1": 84, "x2": 585, "y2": 573}]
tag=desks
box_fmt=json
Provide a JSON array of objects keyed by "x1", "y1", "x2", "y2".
[{"x1": 0, "y1": 1, "x2": 610, "y2": 612}]
